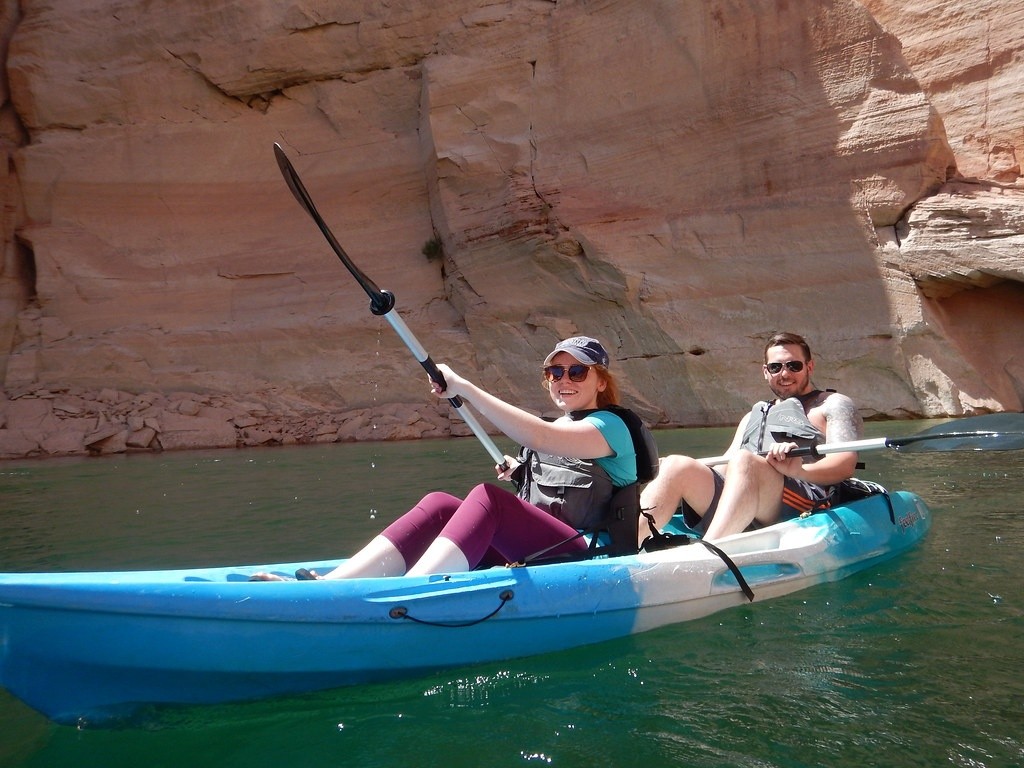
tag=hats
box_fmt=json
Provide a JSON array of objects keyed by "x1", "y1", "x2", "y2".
[{"x1": 545, "y1": 335, "x2": 610, "y2": 370}]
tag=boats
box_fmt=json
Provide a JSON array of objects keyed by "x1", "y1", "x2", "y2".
[{"x1": 0, "y1": 483, "x2": 933, "y2": 726}]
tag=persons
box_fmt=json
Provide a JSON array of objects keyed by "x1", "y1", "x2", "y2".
[
  {"x1": 252, "y1": 336, "x2": 641, "y2": 581},
  {"x1": 637, "y1": 333, "x2": 858, "y2": 552}
]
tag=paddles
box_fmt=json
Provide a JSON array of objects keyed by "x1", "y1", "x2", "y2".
[
  {"x1": 272, "y1": 141, "x2": 521, "y2": 490},
  {"x1": 696, "y1": 412, "x2": 1023, "y2": 467}
]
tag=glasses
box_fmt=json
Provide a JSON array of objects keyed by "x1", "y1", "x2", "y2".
[
  {"x1": 766, "y1": 361, "x2": 806, "y2": 373},
  {"x1": 543, "y1": 363, "x2": 591, "y2": 384}
]
